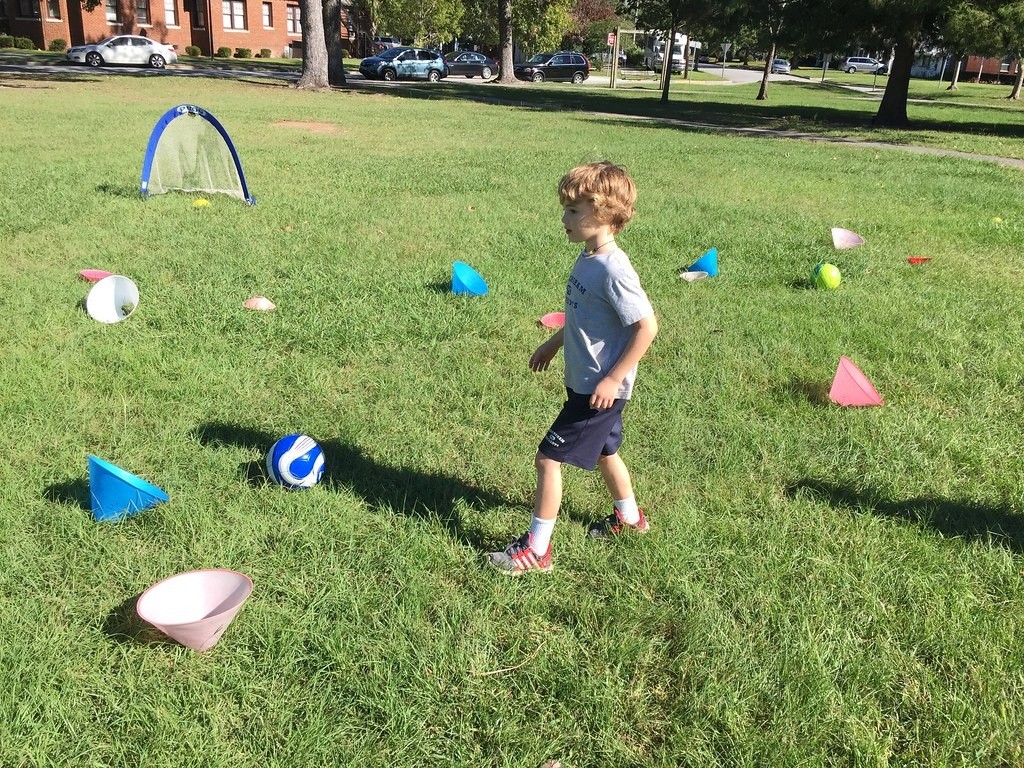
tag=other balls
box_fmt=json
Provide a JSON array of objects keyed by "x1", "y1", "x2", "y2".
[
  {"x1": 265, "y1": 433, "x2": 328, "y2": 491},
  {"x1": 810, "y1": 262, "x2": 842, "y2": 290}
]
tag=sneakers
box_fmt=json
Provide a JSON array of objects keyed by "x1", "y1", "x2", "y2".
[
  {"x1": 487, "y1": 530, "x2": 553, "y2": 577},
  {"x1": 588, "y1": 506, "x2": 651, "y2": 540}
]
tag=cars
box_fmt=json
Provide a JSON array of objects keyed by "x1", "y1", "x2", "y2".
[
  {"x1": 373, "y1": 37, "x2": 401, "y2": 49},
  {"x1": 65, "y1": 34, "x2": 178, "y2": 68},
  {"x1": 444, "y1": 51, "x2": 499, "y2": 80},
  {"x1": 591, "y1": 50, "x2": 627, "y2": 64},
  {"x1": 772, "y1": 59, "x2": 791, "y2": 75},
  {"x1": 842, "y1": 56, "x2": 889, "y2": 76},
  {"x1": 359, "y1": 47, "x2": 449, "y2": 82}
]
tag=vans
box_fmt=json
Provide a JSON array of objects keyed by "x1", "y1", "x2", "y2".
[{"x1": 644, "y1": 40, "x2": 685, "y2": 75}]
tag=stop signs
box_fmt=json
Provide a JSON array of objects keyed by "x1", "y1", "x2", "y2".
[{"x1": 608, "y1": 32, "x2": 615, "y2": 46}]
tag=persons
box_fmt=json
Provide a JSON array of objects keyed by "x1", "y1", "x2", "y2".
[{"x1": 486, "y1": 159, "x2": 659, "y2": 575}]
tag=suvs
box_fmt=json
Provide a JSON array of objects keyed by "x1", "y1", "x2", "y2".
[{"x1": 514, "y1": 53, "x2": 591, "y2": 84}]
